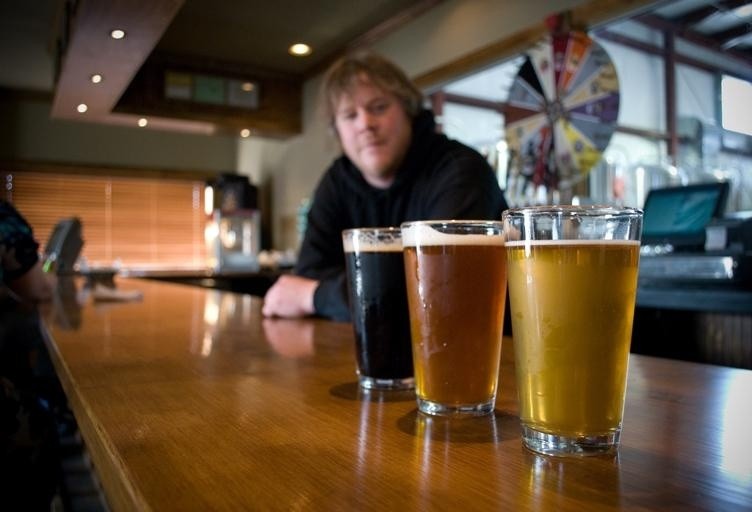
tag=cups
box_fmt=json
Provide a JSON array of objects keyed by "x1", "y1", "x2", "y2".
[
  {"x1": 399, "y1": 219, "x2": 508, "y2": 419},
  {"x1": 341, "y1": 226, "x2": 415, "y2": 390},
  {"x1": 501, "y1": 203, "x2": 644, "y2": 467}
]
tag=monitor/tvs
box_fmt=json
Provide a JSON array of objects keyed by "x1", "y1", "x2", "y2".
[
  {"x1": 637, "y1": 183, "x2": 729, "y2": 248},
  {"x1": 42, "y1": 218, "x2": 80, "y2": 258}
]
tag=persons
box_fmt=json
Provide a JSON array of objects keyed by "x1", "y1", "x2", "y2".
[
  {"x1": 263, "y1": 49, "x2": 523, "y2": 339},
  {"x1": 1, "y1": 198, "x2": 58, "y2": 329}
]
